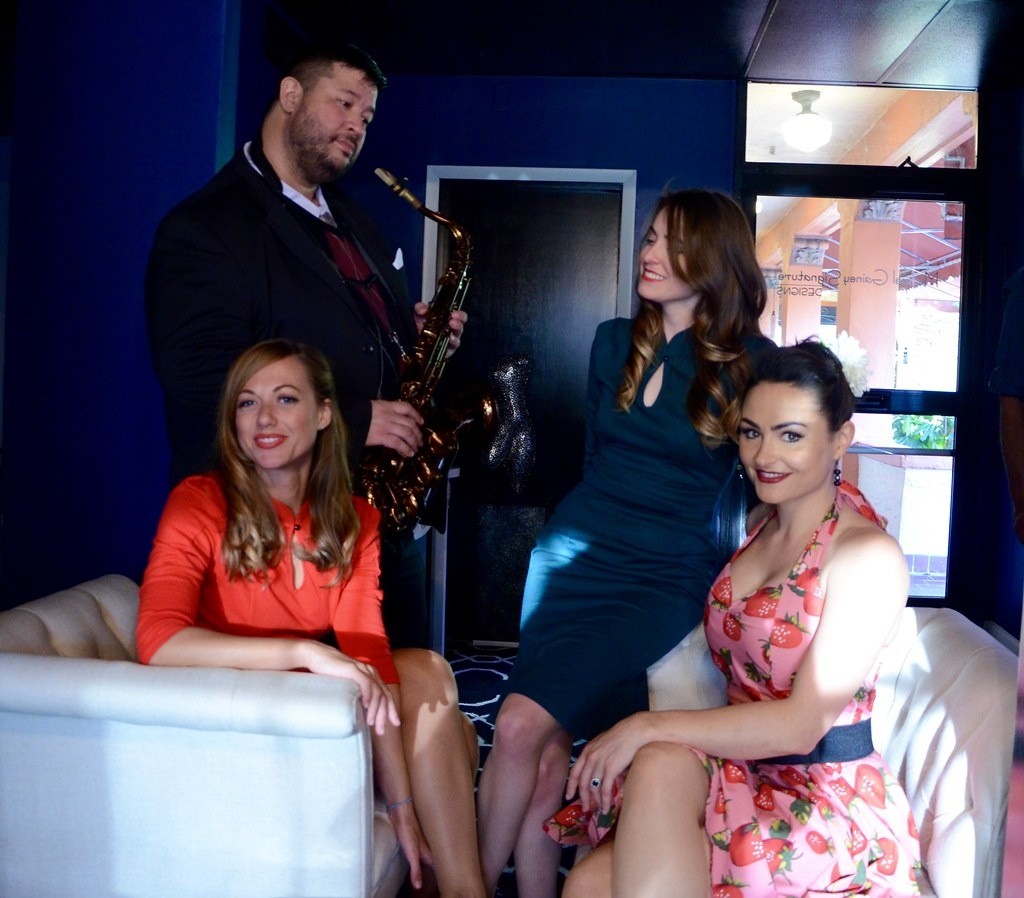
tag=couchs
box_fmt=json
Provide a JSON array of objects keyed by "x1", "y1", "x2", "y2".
[
  {"x1": 649, "y1": 606, "x2": 1019, "y2": 898},
  {"x1": 0, "y1": 574, "x2": 409, "y2": 898}
]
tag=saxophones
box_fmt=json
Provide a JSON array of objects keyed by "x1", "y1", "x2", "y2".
[{"x1": 354, "y1": 165, "x2": 498, "y2": 558}]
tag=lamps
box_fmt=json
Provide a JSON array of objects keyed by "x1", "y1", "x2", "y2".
[{"x1": 788, "y1": 90, "x2": 831, "y2": 152}]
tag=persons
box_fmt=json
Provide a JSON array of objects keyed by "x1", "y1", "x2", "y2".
[
  {"x1": 477, "y1": 187, "x2": 769, "y2": 898},
  {"x1": 144, "y1": 42, "x2": 470, "y2": 650},
  {"x1": 565, "y1": 337, "x2": 911, "y2": 898},
  {"x1": 138, "y1": 337, "x2": 485, "y2": 897}
]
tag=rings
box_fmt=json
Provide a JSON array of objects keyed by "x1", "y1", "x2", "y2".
[{"x1": 589, "y1": 777, "x2": 603, "y2": 789}]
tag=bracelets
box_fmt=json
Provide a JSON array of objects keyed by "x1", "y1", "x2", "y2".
[{"x1": 386, "y1": 795, "x2": 414, "y2": 813}]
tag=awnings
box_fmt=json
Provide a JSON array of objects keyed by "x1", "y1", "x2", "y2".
[{"x1": 820, "y1": 120, "x2": 978, "y2": 291}]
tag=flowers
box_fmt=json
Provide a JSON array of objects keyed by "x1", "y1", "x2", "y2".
[{"x1": 820, "y1": 330, "x2": 871, "y2": 398}]
